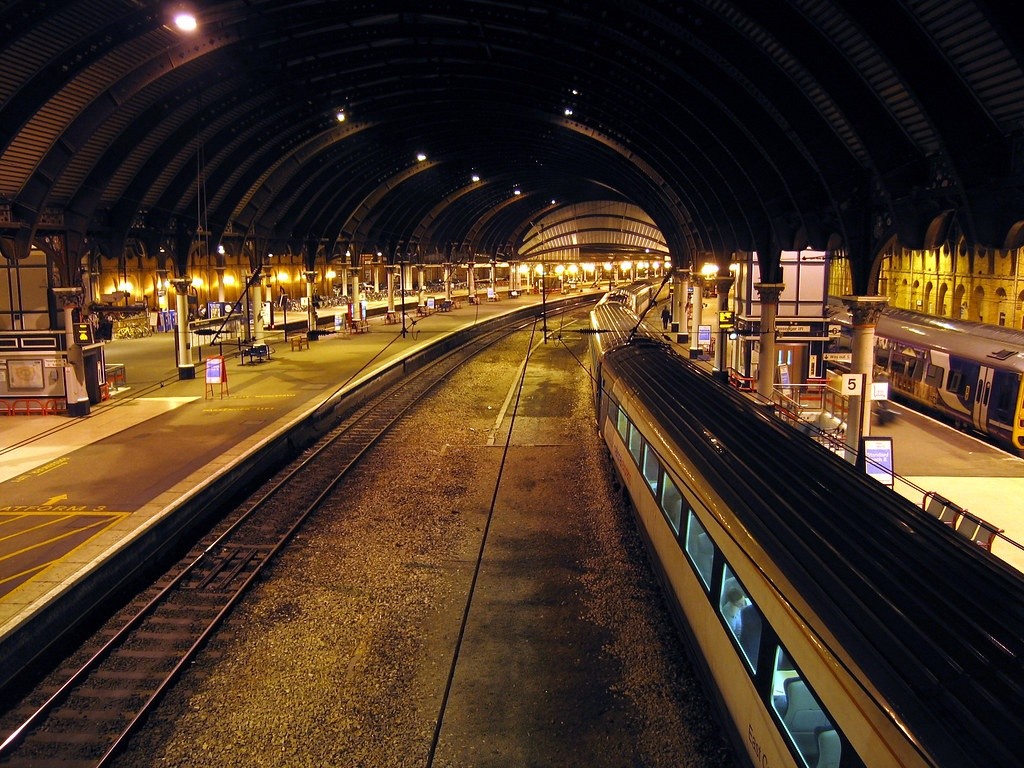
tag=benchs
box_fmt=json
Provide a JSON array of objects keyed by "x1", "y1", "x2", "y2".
[
  {"x1": 241, "y1": 344, "x2": 276, "y2": 364},
  {"x1": 384, "y1": 312, "x2": 401, "y2": 325},
  {"x1": 352, "y1": 320, "x2": 371, "y2": 333},
  {"x1": 290, "y1": 336, "x2": 310, "y2": 351},
  {"x1": 417, "y1": 307, "x2": 430, "y2": 317}
]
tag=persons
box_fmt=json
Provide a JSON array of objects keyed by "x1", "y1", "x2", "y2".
[
  {"x1": 722, "y1": 588, "x2": 752, "y2": 638},
  {"x1": 198, "y1": 304, "x2": 206, "y2": 320},
  {"x1": 661, "y1": 305, "x2": 670, "y2": 329}
]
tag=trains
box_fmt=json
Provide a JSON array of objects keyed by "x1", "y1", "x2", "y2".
[
  {"x1": 587, "y1": 275, "x2": 1024, "y2": 768},
  {"x1": 826, "y1": 295, "x2": 1024, "y2": 457}
]
